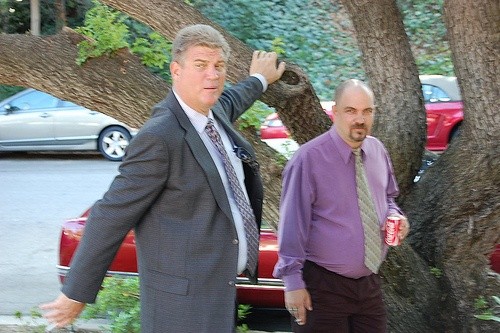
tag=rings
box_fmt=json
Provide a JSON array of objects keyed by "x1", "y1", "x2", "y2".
[
  {"x1": 293, "y1": 308, "x2": 298, "y2": 312},
  {"x1": 288, "y1": 308, "x2": 293, "y2": 311}
]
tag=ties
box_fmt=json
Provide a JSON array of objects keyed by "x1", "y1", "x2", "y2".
[
  {"x1": 352, "y1": 148, "x2": 381, "y2": 274},
  {"x1": 205, "y1": 118, "x2": 259, "y2": 277}
]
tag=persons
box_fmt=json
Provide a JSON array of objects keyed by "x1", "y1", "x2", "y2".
[
  {"x1": 39, "y1": 24, "x2": 286, "y2": 333},
  {"x1": 272, "y1": 79, "x2": 410, "y2": 333}
]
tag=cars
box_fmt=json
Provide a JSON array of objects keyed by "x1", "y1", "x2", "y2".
[
  {"x1": 0, "y1": 88, "x2": 140, "y2": 161},
  {"x1": 56, "y1": 207, "x2": 290, "y2": 317},
  {"x1": 260, "y1": 97, "x2": 336, "y2": 139},
  {"x1": 418, "y1": 74, "x2": 467, "y2": 152}
]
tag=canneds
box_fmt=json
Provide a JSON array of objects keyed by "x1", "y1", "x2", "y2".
[{"x1": 384, "y1": 217, "x2": 402, "y2": 246}]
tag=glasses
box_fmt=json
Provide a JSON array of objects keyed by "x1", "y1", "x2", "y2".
[{"x1": 233, "y1": 145, "x2": 260, "y2": 171}]
